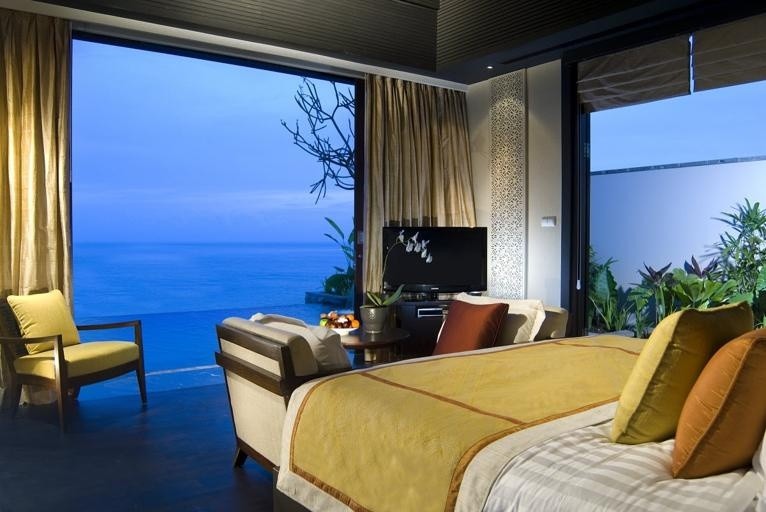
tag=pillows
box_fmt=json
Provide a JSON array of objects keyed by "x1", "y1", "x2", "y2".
[
  {"x1": 222, "y1": 316, "x2": 319, "y2": 377},
  {"x1": 7, "y1": 288, "x2": 81, "y2": 356},
  {"x1": 432, "y1": 291, "x2": 569, "y2": 356},
  {"x1": 608, "y1": 300, "x2": 766, "y2": 480},
  {"x1": 249, "y1": 313, "x2": 351, "y2": 372}
]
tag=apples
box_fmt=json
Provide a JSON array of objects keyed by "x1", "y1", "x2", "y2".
[{"x1": 320, "y1": 311, "x2": 359, "y2": 328}]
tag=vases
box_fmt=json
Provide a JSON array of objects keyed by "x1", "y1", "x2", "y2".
[{"x1": 359, "y1": 304, "x2": 386, "y2": 334}]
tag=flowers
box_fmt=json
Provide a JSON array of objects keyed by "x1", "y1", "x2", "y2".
[{"x1": 366, "y1": 229, "x2": 434, "y2": 307}]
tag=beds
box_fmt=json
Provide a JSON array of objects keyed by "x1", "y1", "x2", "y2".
[{"x1": 276, "y1": 334, "x2": 766, "y2": 512}]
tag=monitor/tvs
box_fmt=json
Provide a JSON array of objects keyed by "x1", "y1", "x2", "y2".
[{"x1": 381, "y1": 226, "x2": 488, "y2": 301}]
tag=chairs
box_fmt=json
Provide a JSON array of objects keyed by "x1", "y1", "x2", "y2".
[
  {"x1": 214, "y1": 323, "x2": 352, "y2": 473},
  {"x1": 1, "y1": 298, "x2": 147, "y2": 433}
]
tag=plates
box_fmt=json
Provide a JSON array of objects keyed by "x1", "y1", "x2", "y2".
[{"x1": 329, "y1": 328, "x2": 356, "y2": 336}]
